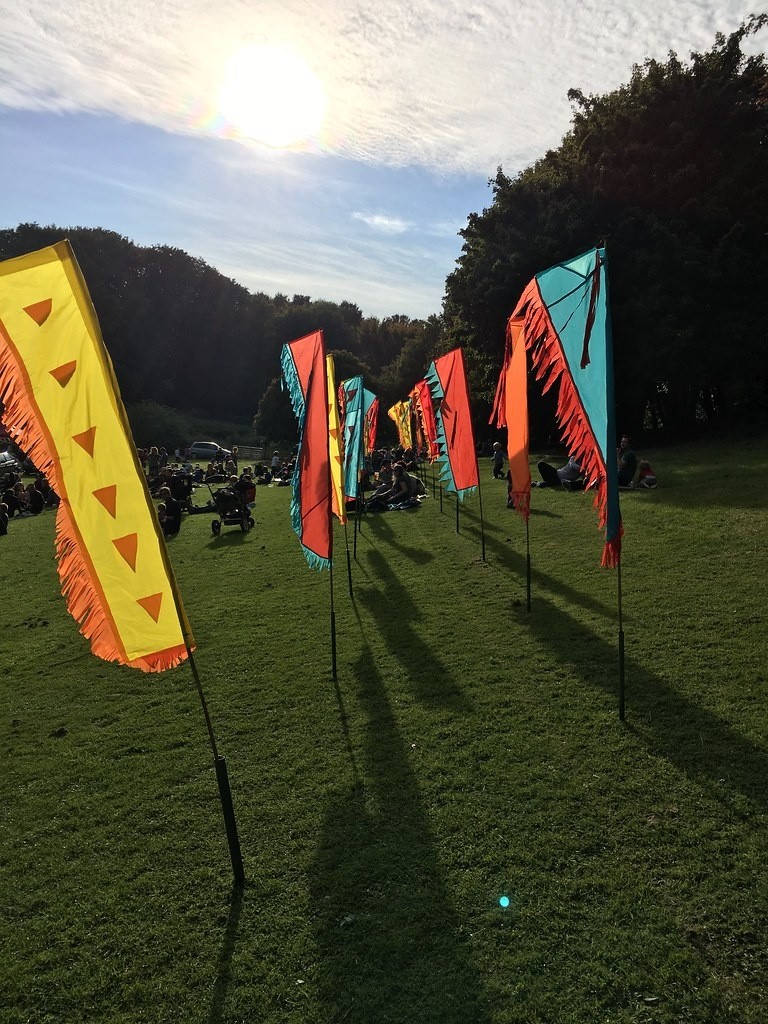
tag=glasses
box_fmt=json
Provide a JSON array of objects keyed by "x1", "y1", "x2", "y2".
[{"x1": 160, "y1": 492, "x2": 166, "y2": 496}]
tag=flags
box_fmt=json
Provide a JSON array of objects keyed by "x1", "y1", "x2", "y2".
[
  {"x1": 510, "y1": 247, "x2": 624, "y2": 567},
  {"x1": 338, "y1": 375, "x2": 379, "y2": 503},
  {"x1": 326, "y1": 353, "x2": 348, "y2": 525},
  {"x1": 0, "y1": 239, "x2": 197, "y2": 673},
  {"x1": 489, "y1": 316, "x2": 531, "y2": 521},
  {"x1": 388, "y1": 379, "x2": 440, "y2": 465},
  {"x1": 424, "y1": 347, "x2": 480, "y2": 503},
  {"x1": 281, "y1": 330, "x2": 333, "y2": 572}
]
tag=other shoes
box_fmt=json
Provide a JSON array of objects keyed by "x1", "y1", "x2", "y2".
[{"x1": 561, "y1": 479, "x2": 571, "y2": 491}]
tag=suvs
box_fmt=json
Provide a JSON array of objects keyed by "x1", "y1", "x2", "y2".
[
  {"x1": 190, "y1": 441, "x2": 231, "y2": 460},
  {"x1": 0, "y1": 452, "x2": 20, "y2": 476}
]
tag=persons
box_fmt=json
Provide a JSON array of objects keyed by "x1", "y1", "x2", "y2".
[
  {"x1": 491, "y1": 442, "x2": 508, "y2": 479},
  {"x1": 507, "y1": 470, "x2": 513, "y2": 508},
  {"x1": 0, "y1": 437, "x2": 60, "y2": 536},
  {"x1": 537, "y1": 435, "x2": 659, "y2": 488},
  {"x1": 345, "y1": 442, "x2": 428, "y2": 512},
  {"x1": 137, "y1": 443, "x2": 300, "y2": 536}
]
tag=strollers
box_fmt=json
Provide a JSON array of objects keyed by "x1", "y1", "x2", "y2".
[
  {"x1": 0, "y1": 472, "x2": 21, "y2": 494},
  {"x1": 203, "y1": 476, "x2": 255, "y2": 534}
]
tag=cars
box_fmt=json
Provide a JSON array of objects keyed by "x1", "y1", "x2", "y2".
[
  {"x1": 474, "y1": 434, "x2": 491, "y2": 457},
  {"x1": 23, "y1": 456, "x2": 40, "y2": 475}
]
